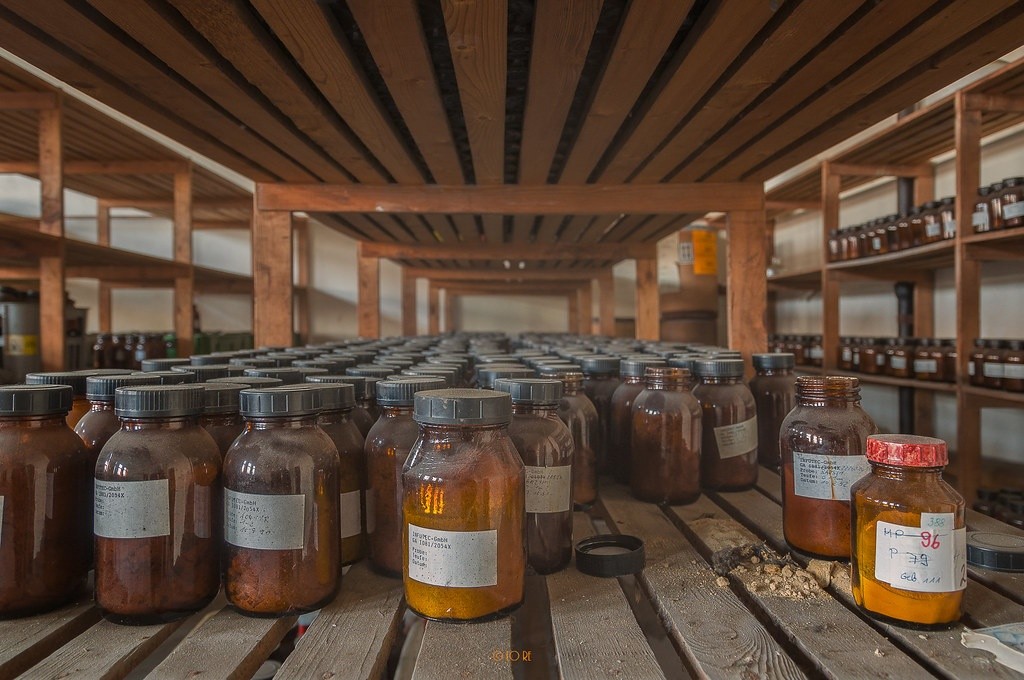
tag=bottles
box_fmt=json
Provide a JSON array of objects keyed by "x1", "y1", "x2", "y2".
[
  {"x1": 470, "y1": 331, "x2": 761, "y2": 574},
  {"x1": 746, "y1": 352, "x2": 799, "y2": 469},
  {"x1": 768, "y1": 334, "x2": 823, "y2": 368},
  {"x1": 837, "y1": 335, "x2": 956, "y2": 382},
  {"x1": 967, "y1": 338, "x2": 1024, "y2": 393},
  {"x1": 850, "y1": 431, "x2": 968, "y2": 633},
  {"x1": 0, "y1": 353, "x2": 225, "y2": 626},
  {"x1": 91, "y1": 328, "x2": 176, "y2": 368},
  {"x1": 200, "y1": 345, "x2": 341, "y2": 616},
  {"x1": 970, "y1": 174, "x2": 1024, "y2": 234},
  {"x1": 778, "y1": 375, "x2": 878, "y2": 562},
  {"x1": 826, "y1": 197, "x2": 957, "y2": 264},
  {"x1": 321, "y1": 329, "x2": 527, "y2": 624}
]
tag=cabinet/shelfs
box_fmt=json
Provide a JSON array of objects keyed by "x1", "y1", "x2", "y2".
[{"x1": 0, "y1": 0, "x2": 1024, "y2": 680}]
